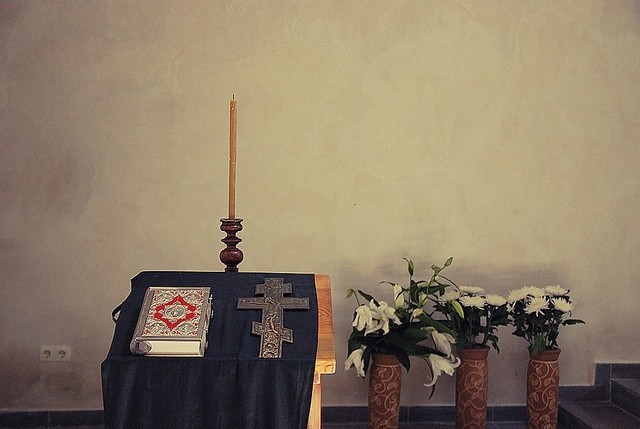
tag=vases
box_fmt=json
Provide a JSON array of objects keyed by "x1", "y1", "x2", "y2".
[
  {"x1": 454, "y1": 343, "x2": 491, "y2": 429},
  {"x1": 525, "y1": 345, "x2": 561, "y2": 429},
  {"x1": 366, "y1": 352, "x2": 401, "y2": 429}
]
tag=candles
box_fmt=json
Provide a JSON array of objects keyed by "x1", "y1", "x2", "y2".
[{"x1": 228, "y1": 94, "x2": 239, "y2": 220}]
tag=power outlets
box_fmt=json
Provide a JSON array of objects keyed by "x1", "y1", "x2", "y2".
[{"x1": 38, "y1": 345, "x2": 72, "y2": 361}]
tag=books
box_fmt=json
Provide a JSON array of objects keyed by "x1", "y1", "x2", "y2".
[{"x1": 130, "y1": 286, "x2": 213, "y2": 358}]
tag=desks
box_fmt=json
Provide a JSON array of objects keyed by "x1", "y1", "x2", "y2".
[{"x1": 100, "y1": 271, "x2": 338, "y2": 429}]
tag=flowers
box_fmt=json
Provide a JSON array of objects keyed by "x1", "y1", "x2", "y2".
[
  {"x1": 437, "y1": 285, "x2": 511, "y2": 353},
  {"x1": 508, "y1": 284, "x2": 586, "y2": 354},
  {"x1": 343, "y1": 257, "x2": 464, "y2": 400}
]
